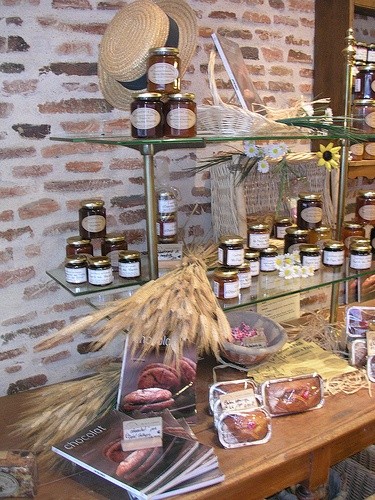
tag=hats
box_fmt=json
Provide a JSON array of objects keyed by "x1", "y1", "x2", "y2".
[{"x1": 97, "y1": 0, "x2": 198, "y2": 111}]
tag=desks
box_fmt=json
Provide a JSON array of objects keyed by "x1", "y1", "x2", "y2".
[{"x1": 0, "y1": 356, "x2": 375, "y2": 500}]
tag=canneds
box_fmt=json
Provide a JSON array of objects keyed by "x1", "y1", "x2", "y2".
[
  {"x1": 213, "y1": 189, "x2": 345, "y2": 300},
  {"x1": 130, "y1": 46, "x2": 198, "y2": 140},
  {"x1": 341, "y1": 42, "x2": 375, "y2": 270},
  {"x1": 65, "y1": 199, "x2": 142, "y2": 286},
  {"x1": 157, "y1": 190, "x2": 177, "y2": 243}
]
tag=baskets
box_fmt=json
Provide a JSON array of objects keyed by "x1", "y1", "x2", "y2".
[
  {"x1": 210, "y1": 152, "x2": 336, "y2": 247},
  {"x1": 218, "y1": 310, "x2": 289, "y2": 365},
  {"x1": 194, "y1": 44, "x2": 316, "y2": 136}
]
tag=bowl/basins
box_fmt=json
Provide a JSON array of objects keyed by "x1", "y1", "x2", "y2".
[{"x1": 219, "y1": 309, "x2": 288, "y2": 366}]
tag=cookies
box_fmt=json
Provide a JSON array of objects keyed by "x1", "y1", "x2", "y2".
[
  {"x1": 104, "y1": 426, "x2": 186, "y2": 479},
  {"x1": 122, "y1": 358, "x2": 196, "y2": 411},
  {"x1": 350, "y1": 275, "x2": 375, "y2": 296}
]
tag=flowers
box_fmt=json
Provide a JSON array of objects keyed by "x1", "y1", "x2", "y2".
[
  {"x1": 184, "y1": 102, "x2": 368, "y2": 217},
  {"x1": 274, "y1": 250, "x2": 316, "y2": 278}
]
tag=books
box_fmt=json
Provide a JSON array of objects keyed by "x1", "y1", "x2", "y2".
[{"x1": 51, "y1": 334, "x2": 224, "y2": 500}]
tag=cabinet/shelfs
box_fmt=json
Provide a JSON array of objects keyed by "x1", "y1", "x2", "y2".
[
  {"x1": 310, "y1": 0, "x2": 375, "y2": 306},
  {"x1": 43, "y1": 137, "x2": 375, "y2": 335}
]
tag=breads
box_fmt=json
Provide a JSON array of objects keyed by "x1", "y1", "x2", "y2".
[
  {"x1": 215, "y1": 376, "x2": 320, "y2": 441},
  {"x1": 350, "y1": 311, "x2": 375, "y2": 378}
]
